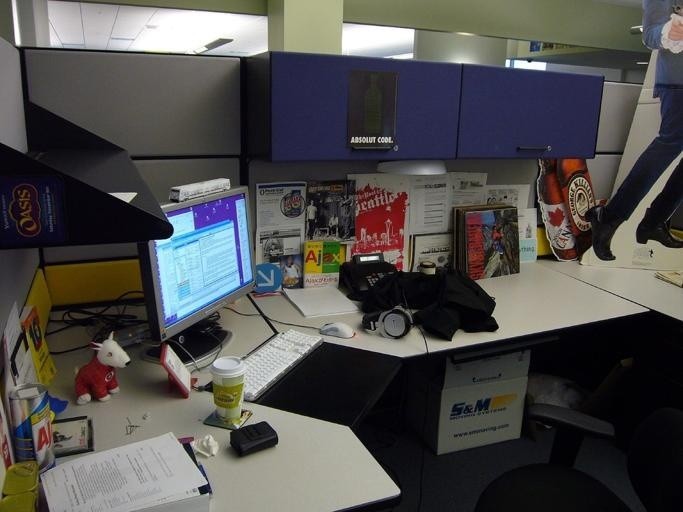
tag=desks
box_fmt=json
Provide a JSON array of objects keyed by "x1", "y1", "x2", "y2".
[
  {"x1": 537, "y1": 254, "x2": 683, "y2": 370},
  {"x1": 244, "y1": 261, "x2": 650, "y2": 427},
  {"x1": 38, "y1": 295, "x2": 401, "y2": 512}
]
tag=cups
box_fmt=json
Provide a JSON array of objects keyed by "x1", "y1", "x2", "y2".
[{"x1": 208, "y1": 356, "x2": 246, "y2": 423}]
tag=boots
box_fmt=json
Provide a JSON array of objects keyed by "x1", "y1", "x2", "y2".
[
  {"x1": 636, "y1": 208, "x2": 683, "y2": 248},
  {"x1": 585, "y1": 205, "x2": 615, "y2": 260}
]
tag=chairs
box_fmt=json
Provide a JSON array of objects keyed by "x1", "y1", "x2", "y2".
[{"x1": 473, "y1": 360, "x2": 683, "y2": 512}]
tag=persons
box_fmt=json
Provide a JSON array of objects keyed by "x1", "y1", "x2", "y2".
[
  {"x1": 583, "y1": 1, "x2": 683, "y2": 263},
  {"x1": 268, "y1": 243, "x2": 279, "y2": 263},
  {"x1": 282, "y1": 256, "x2": 302, "y2": 287},
  {"x1": 306, "y1": 197, "x2": 352, "y2": 240}
]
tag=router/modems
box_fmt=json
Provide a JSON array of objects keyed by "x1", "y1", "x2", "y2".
[{"x1": 104, "y1": 328, "x2": 141, "y2": 347}]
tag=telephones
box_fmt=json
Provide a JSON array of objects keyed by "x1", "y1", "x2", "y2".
[{"x1": 338, "y1": 254, "x2": 398, "y2": 300}]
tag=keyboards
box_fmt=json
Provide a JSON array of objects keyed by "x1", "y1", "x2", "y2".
[{"x1": 212, "y1": 329, "x2": 323, "y2": 401}]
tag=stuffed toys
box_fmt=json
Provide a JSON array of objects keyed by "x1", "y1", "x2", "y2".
[{"x1": 74, "y1": 332, "x2": 132, "y2": 404}]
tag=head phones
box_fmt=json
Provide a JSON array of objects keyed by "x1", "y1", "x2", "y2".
[{"x1": 362, "y1": 306, "x2": 414, "y2": 339}]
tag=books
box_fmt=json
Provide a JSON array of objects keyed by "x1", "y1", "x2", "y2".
[
  {"x1": 20, "y1": 304, "x2": 59, "y2": 386},
  {"x1": 50, "y1": 414, "x2": 95, "y2": 458},
  {"x1": 451, "y1": 203, "x2": 520, "y2": 281},
  {"x1": 302, "y1": 239, "x2": 346, "y2": 288},
  {"x1": 38, "y1": 431, "x2": 214, "y2": 512}
]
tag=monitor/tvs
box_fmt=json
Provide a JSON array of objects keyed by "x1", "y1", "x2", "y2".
[{"x1": 137, "y1": 186, "x2": 256, "y2": 366}]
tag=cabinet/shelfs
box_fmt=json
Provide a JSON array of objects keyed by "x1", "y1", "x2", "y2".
[
  {"x1": 457, "y1": 63, "x2": 605, "y2": 160},
  {"x1": 240, "y1": 51, "x2": 463, "y2": 163}
]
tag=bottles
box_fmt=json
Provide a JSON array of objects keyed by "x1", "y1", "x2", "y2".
[{"x1": 536, "y1": 158, "x2": 596, "y2": 261}]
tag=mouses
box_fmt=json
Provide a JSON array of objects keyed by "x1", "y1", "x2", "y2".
[{"x1": 320, "y1": 322, "x2": 355, "y2": 339}]
